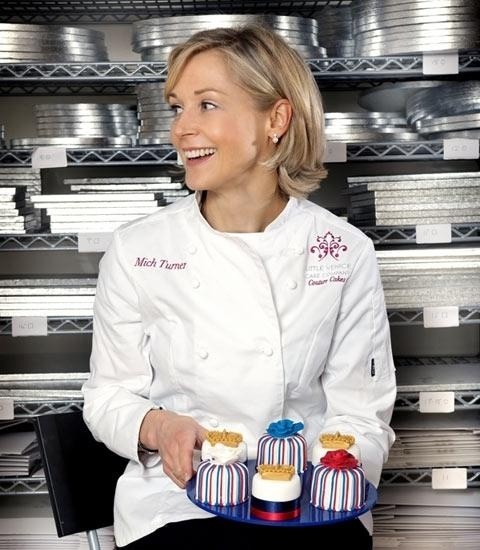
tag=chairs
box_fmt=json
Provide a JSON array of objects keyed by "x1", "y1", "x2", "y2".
[{"x1": 33, "y1": 411, "x2": 129, "y2": 548}]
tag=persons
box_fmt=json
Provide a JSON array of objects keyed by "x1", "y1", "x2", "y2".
[{"x1": 81, "y1": 26, "x2": 396, "y2": 547}]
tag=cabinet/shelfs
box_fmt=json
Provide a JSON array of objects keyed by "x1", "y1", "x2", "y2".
[{"x1": 0, "y1": 1, "x2": 480, "y2": 549}]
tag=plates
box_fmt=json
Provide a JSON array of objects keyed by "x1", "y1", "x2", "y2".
[{"x1": 186, "y1": 458, "x2": 379, "y2": 527}]
